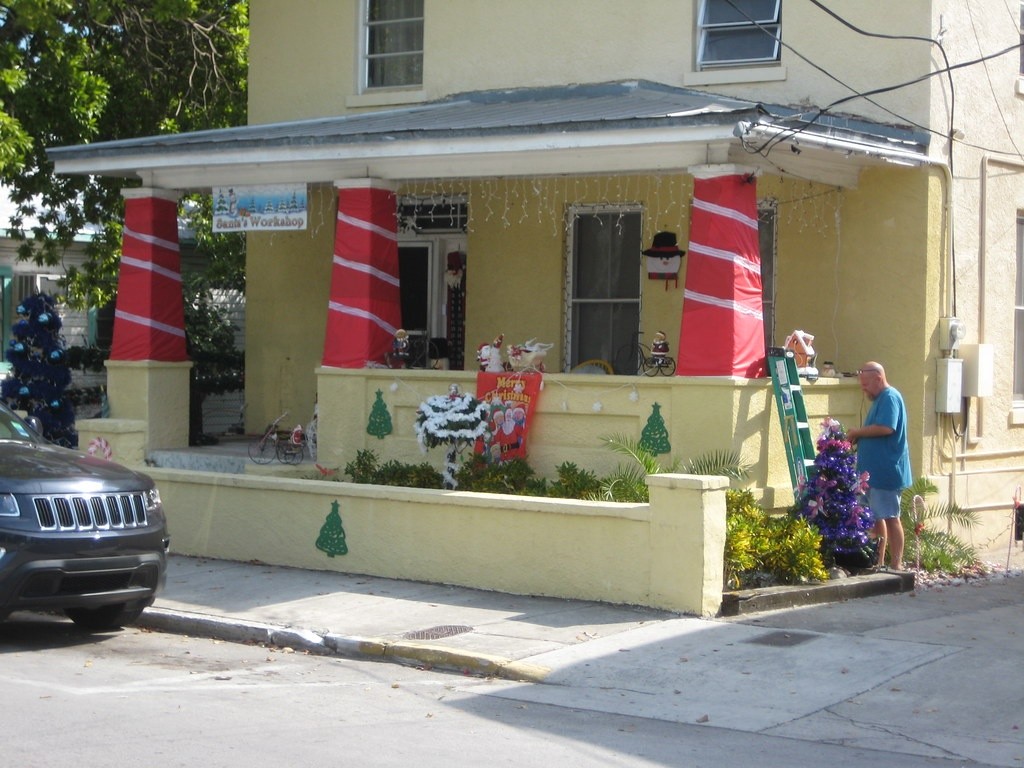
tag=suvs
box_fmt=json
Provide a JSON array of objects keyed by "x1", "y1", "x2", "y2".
[{"x1": 0, "y1": 401, "x2": 171, "y2": 630}]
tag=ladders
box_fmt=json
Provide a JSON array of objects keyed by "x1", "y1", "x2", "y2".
[{"x1": 767, "y1": 345, "x2": 817, "y2": 507}]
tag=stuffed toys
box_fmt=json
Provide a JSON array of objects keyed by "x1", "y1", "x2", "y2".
[
  {"x1": 650, "y1": 331, "x2": 670, "y2": 365},
  {"x1": 448, "y1": 383, "x2": 459, "y2": 399},
  {"x1": 476, "y1": 333, "x2": 505, "y2": 372},
  {"x1": 391, "y1": 327, "x2": 410, "y2": 367}
]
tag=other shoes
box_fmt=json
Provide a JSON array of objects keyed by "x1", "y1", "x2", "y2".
[
  {"x1": 881, "y1": 567, "x2": 903, "y2": 575},
  {"x1": 865, "y1": 565, "x2": 886, "y2": 572}
]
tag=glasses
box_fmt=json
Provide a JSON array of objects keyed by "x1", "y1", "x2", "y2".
[{"x1": 855, "y1": 368, "x2": 878, "y2": 375}]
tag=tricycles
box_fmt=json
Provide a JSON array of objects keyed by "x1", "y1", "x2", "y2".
[
  {"x1": 616, "y1": 329, "x2": 678, "y2": 377},
  {"x1": 248, "y1": 423, "x2": 307, "y2": 465}
]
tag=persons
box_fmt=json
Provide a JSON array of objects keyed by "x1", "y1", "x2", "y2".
[{"x1": 846, "y1": 361, "x2": 913, "y2": 570}]
